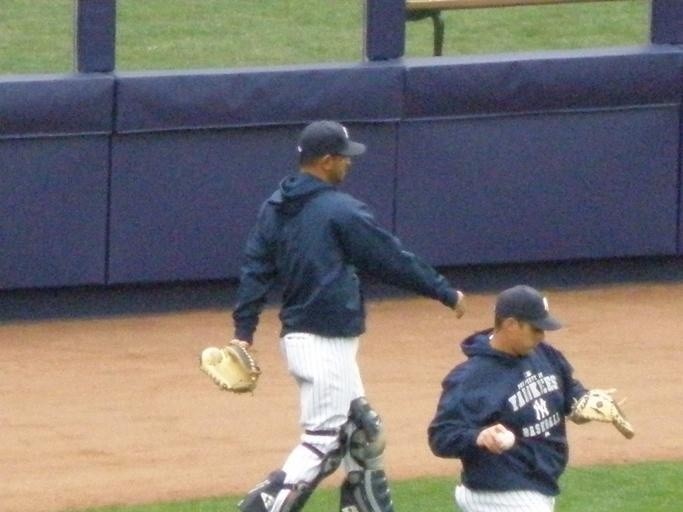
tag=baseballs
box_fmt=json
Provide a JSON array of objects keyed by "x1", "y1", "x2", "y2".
[{"x1": 497, "y1": 432, "x2": 515, "y2": 451}]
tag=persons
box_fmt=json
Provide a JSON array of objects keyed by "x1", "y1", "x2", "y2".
[
  {"x1": 232, "y1": 118, "x2": 466, "y2": 512},
  {"x1": 427, "y1": 286, "x2": 633, "y2": 512}
]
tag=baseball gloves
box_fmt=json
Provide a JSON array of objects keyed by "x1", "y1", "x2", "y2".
[
  {"x1": 569, "y1": 388, "x2": 636, "y2": 440},
  {"x1": 200, "y1": 340, "x2": 261, "y2": 393}
]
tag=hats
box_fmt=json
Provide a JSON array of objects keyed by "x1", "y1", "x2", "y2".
[
  {"x1": 293, "y1": 119, "x2": 368, "y2": 157},
  {"x1": 492, "y1": 284, "x2": 564, "y2": 332}
]
